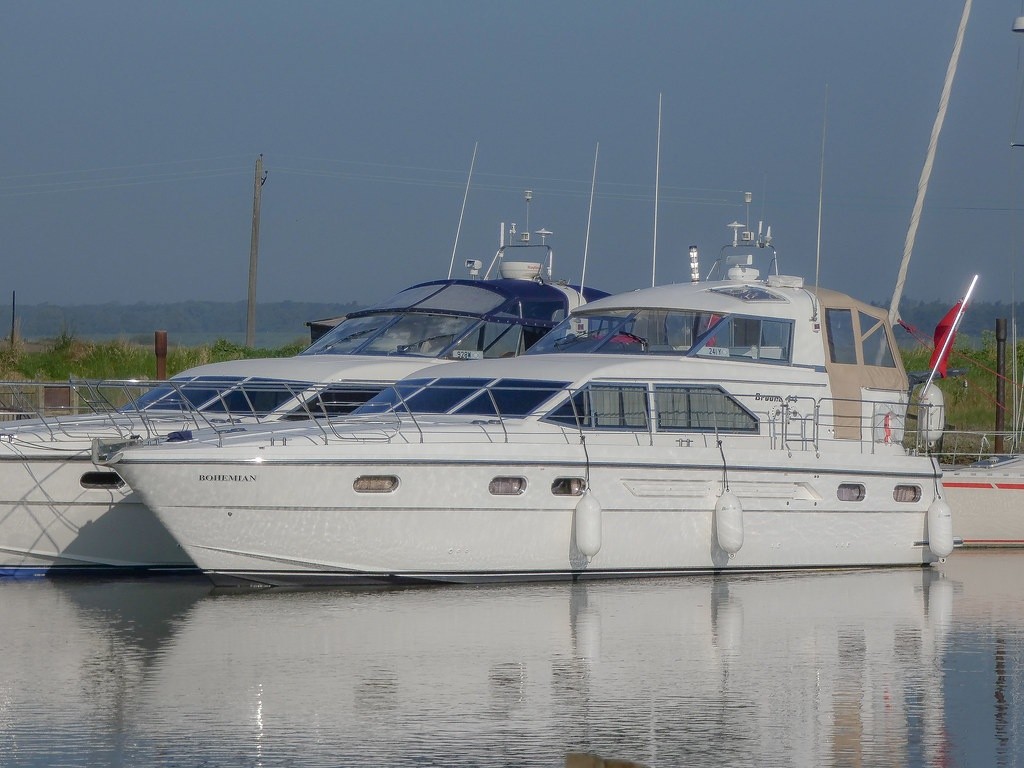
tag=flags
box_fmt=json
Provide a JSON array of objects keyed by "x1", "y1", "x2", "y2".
[{"x1": 928, "y1": 294, "x2": 971, "y2": 379}]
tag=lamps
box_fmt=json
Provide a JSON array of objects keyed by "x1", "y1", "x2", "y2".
[{"x1": 465, "y1": 259, "x2": 482, "y2": 270}]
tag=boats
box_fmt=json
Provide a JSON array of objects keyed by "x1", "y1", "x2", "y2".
[
  {"x1": 0, "y1": 93, "x2": 785, "y2": 578},
  {"x1": 938, "y1": 453, "x2": 1024, "y2": 548},
  {"x1": 91, "y1": 189, "x2": 954, "y2": 588}
]
